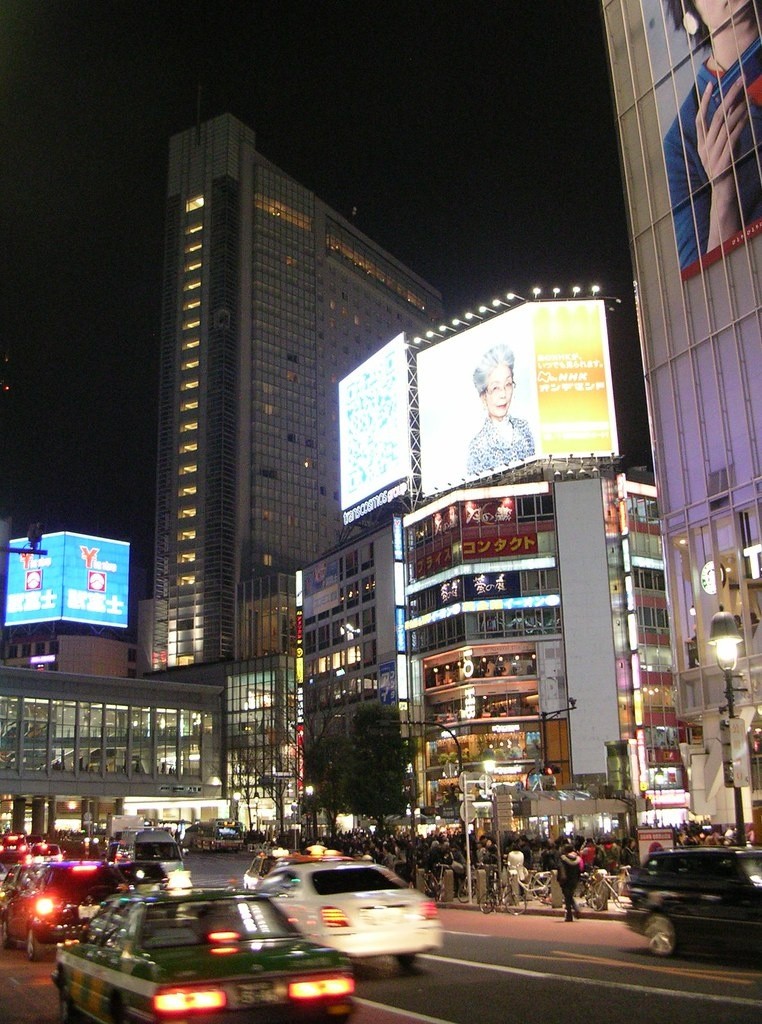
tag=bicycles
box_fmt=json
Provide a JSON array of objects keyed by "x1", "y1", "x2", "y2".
[{"x1": 424, "y1": 863, "x2": 634, "y2": 915}]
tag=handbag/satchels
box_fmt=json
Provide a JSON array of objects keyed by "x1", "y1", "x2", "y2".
[{"x1": 558, "y1": 862, "x2": 577, "y2": 888}]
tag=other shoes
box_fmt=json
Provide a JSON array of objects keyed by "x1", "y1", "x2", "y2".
[
  {"x1": 574, "y1": 906, "x2": 580, "y2": 919},
  {"x1": 564, "y1": 912, "x2": 573, "y2": 922}
]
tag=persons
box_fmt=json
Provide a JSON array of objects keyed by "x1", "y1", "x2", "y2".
[
  {"x1": 40, "y1": 760, "x2": 176, "y2": 774},
  {"x1": 661, "y1": 0, "x2": 762, "y2": 270},
  {"x1": 468, "y1": 344, "x2": 535, "y2": 476},
  {"x1": 244, "y1": 818, "x2": 755, "y2": 922}
]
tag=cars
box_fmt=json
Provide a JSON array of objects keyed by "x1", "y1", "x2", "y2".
[
  {"x1": 621, "y1": 844, "x2": 762, "y2": 962},
  {"x1": 243, "y1": 848, "x2": 358, "y2": 891},
  {"x1": 0, "y1": 815, "x2": 194, "y2": 963},
  {"x1": 50, "y1": 884, "x2": 355, "y2": 1024},
  {"x1": 253, "y1": 861, "x2": 444, "y2": 978}
]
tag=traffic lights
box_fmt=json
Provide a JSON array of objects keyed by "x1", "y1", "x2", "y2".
[
  {"x1": 709, "y1": 602, "x2": 748, "y2": 847},
  {"x1": 541, "y1": 766, "x2": 561, "y2": 778}
]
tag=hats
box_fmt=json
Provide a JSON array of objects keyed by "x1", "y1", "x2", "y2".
[{"x1": 725, "y1": 829, "x2": 734, "y2": 840}]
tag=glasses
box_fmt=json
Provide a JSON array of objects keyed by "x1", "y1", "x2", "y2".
[{"x1": 485, "y1": 381, "x2": 516, "y2": 395}]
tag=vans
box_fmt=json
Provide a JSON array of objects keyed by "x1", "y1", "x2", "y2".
[{"x1": 193, "y1": 817, "x2": 246, "y2": 853}]
